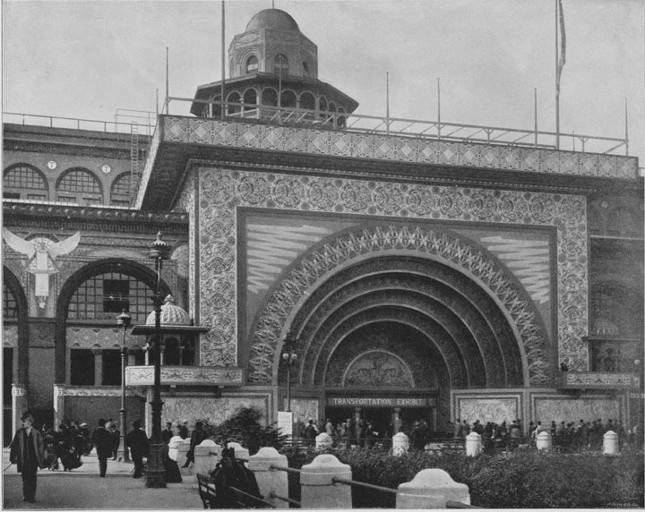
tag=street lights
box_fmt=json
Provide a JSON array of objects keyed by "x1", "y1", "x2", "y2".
[
  {"x1": 282, "y1": 353, "x2": 298, "y2": 412},
  {"x1": 116, "y1": 307, "x2": 131, "y2": 463},
  {"x1": 146, "y1": 230, "x2": 171, "y2": 488}
]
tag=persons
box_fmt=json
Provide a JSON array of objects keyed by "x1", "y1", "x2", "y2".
[
  {"x1": 23, "y1": 238, "x2": 59, "y2": 309},
  {"x1": 301, "y1": 414, "x2": 437, "y2": 449},
  {"x1": 445, "y1": 416, "x2": 627, "y2": 450},
  {"x1": 214, "y1": 448, "x2": 263, "y2": 504},
  {"x1": 40, "y1": 419, "x2": 208, "y2": 479},
  {"x1": 9, "y1": 411, "x2": 53, "y2": 504}
]
